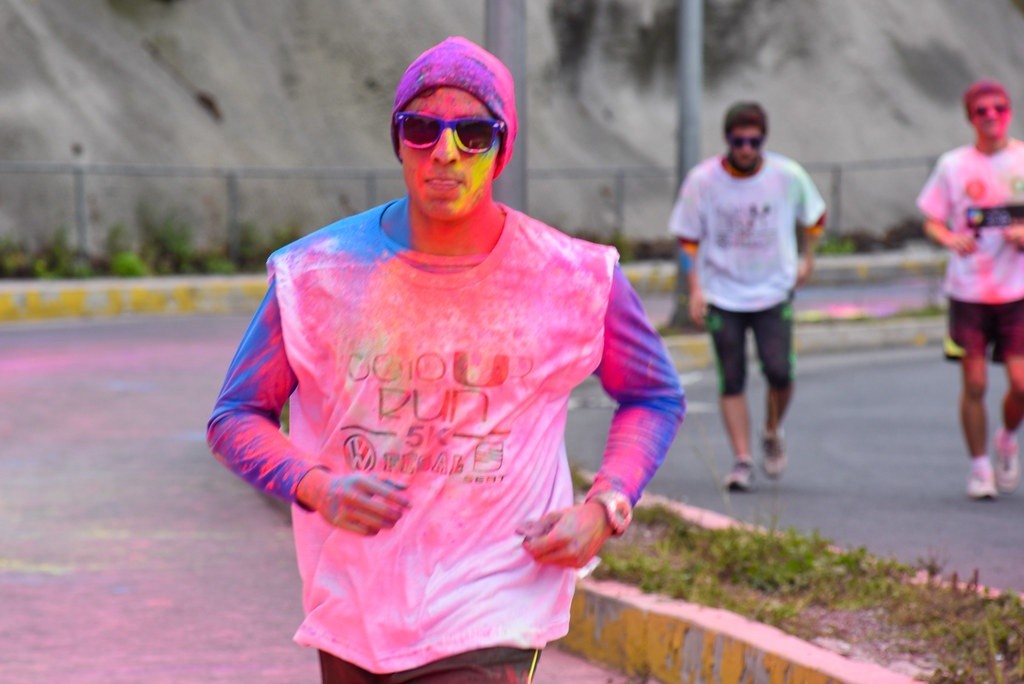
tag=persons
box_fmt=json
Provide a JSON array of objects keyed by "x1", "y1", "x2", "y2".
[
  {"x1": 668, "y1": 101, "x2": 828, "y2": 494},
  {"x1": 916, "y1": 80, "x2": 1024, "y2": 501},
  {"x1": 205, "y1": 37, "x2": 685, "y2": 684}
]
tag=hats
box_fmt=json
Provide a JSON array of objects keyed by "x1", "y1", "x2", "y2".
[
  {"x1": 391, "y1": 35, "x2": 518, "y2": 180},
  {"x1": 965, "y1": 79, "x2": 1011, "y2": 113}
]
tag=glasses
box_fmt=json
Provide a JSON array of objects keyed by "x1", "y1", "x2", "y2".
[
  {"x1": 972, "y1": 105, "x2": 1006, "y2": 119},
  {"x1": 395, "y1": 111, "x2": 504, "y2": 153},
  {"x1": 728, "y1": 136, "x2": 763, "y2": 150}
]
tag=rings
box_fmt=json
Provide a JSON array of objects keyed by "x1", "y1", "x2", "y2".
[{"x1": 351, "y1": 492, "x2": 359, "y2": 500}]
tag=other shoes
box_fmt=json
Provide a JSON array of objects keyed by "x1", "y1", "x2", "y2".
[
  {"x1": 760, "y1": 429, "x2": 786, "y2": 478},
  {"x1": 993, "y1": 431, "x2": 1019, "y2": 493},
  {"x1": 967, "y1": 468, "x2": 996, "y2": 499},
  {"x1": 726, "y1": 459, "x2": 756, "y2": 490}
]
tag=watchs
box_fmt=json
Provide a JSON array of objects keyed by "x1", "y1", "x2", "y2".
[{"x1": 587, "y1": 490, "x2": 634, "y2": 539}]
